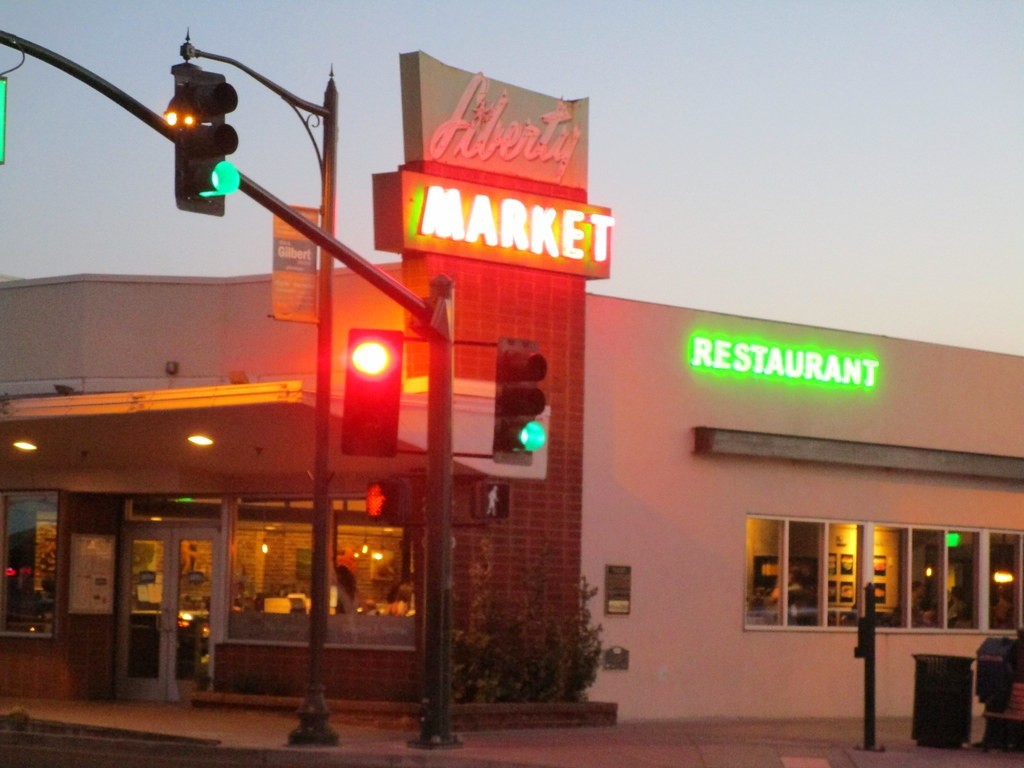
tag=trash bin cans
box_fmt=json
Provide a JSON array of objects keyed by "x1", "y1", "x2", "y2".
[{"x1": 910, "y1": 650, "x2": 975, "y2": 748}]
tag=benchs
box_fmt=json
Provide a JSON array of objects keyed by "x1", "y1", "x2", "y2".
[{"x1": 981, "y1": 683, "x2": 1024, "y2": 753}]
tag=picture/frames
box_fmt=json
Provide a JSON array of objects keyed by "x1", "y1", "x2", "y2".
[
  {"x1": 827, "y1": 580, "x2": 837, "y2": 603},
  {"x1": 839, "y1": 581, "x2": 854, "y2": 603},
  {"x1": 873, "y1": 583, "x2": 886, "y2": 604},
  {"x1": 828, "y1": 552, "x2": 837, "y2": 574},
  {"x1": 828, "y1": 610, "x2": 837, "y2": 626},
  {"x1": 873, "y1": 554, "x2": 886, "y2": 577},
  {"x1": 840, "y1": 554, "x2": 853, "y2": 575},
  {"x1": 839, "y1": 610, "x2": 850, "y2": 627}
]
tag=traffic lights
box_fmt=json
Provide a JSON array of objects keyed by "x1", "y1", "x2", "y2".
[
  {"x1": 174, "y1": 66, "x2": 241, "y2": 215},
  {"x1": 491, "y1": 334, "x2": 548, "y2": 468},
  {"x1": 468, "y1": 481, "x2": 512, "y2": 520},
  {"x1": 339, "y1": 326, "x2": 405, "y2": 458},
  {"x1": 362, "y1": 482, "x2": 414, "y2": 527}
]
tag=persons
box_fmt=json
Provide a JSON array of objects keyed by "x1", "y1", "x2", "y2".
[
  {"x1": 947, "y1": 585, "x2": 973, "y2": 627},
  {"x1": 889, "y1": 580, "x2": 924, "y2": 628}
]
